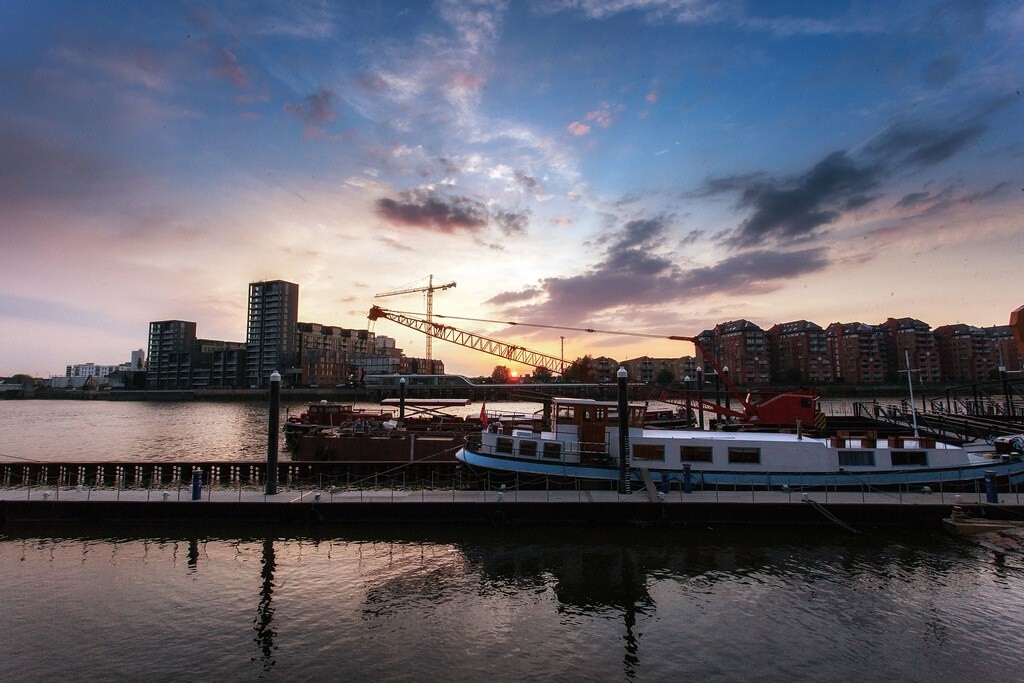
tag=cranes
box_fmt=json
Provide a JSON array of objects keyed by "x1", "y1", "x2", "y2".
[{"x1": 373, "y1": 273, "x2": 456, "y2": 374}]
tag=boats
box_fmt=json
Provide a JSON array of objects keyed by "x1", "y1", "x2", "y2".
[
  {"x1": 287, "y1": 399, "x2": 398, "y2": 425},
  {"x1": 282, "y1": 394, "x2": 697, "y2": 461},
  {"x1": 452, "y1": 349, "x2": 1024, "y2": 488}
]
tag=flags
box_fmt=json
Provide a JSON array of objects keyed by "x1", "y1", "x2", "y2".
[{"x1": 479, "y1": 401, "x2": 489, "y2": 429}]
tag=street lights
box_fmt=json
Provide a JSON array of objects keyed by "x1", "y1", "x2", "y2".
[{"x1": 561, "y1": 335, "x2": 565, "y2": 384}]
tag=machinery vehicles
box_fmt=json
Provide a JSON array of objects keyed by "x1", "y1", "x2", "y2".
[{"x1": 368, "y1": 301, "x2": 826, "y2": 431}]
